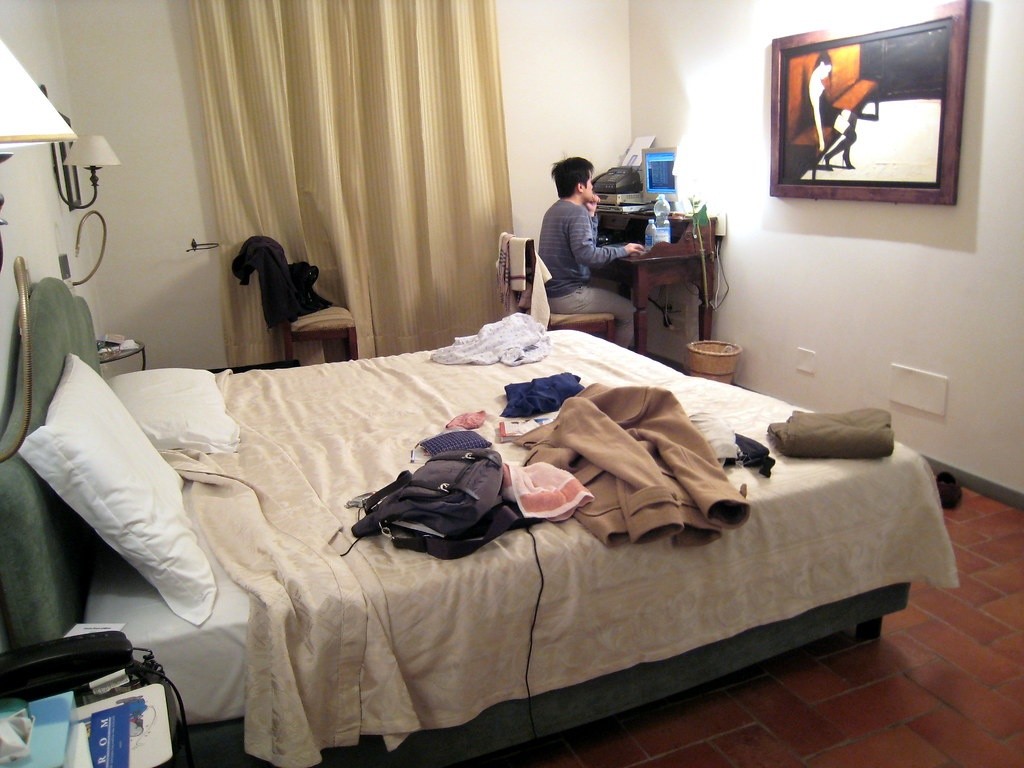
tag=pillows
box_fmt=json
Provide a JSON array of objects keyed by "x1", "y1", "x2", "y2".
[
  {"x1": 104, "y1": 364, "x2": 241, "y2": 453},
  {"x1": 18, "y1": 350, "x2": 218, "y2": 628}
]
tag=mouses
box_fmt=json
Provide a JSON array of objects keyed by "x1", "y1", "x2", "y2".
[{"x1": 630, "y1": 248, "x2": 643, "y2": 257}]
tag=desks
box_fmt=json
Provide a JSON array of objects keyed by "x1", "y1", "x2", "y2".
[
  {"x1": 96, "y1": 341, "x2": 147, "y2": 381},
  {"x1": 597, "y1": 212, "x2": 716, "y2": 367}
]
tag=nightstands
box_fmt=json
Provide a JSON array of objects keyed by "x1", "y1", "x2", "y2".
[{"x1": 76, "y1": 666, "x2": 177, "y2": 768}]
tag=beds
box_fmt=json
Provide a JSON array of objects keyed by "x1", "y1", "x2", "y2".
[{"x1": 0, "y1": 245, "x2": 957, "y2": 768}]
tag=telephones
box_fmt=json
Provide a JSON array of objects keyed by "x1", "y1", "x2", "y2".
[{"x1": 0, "y1": 630, "x2": 135, "y2": 703}]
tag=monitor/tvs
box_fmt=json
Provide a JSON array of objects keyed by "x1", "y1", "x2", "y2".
[{"x1": 641, "y1": 147, "x2": 681, "y2": 212}]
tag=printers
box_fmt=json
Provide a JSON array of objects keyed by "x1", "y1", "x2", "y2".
[{"x1": 589, "y1": 167, "x2": 643, "y2": 194}]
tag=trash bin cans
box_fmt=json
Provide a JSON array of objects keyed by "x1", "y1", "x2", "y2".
[{"x1": 685, "y1": 339, "x2": 744, "y2": 384}]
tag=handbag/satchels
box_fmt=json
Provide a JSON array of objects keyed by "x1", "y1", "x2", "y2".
[{"x1": 353, "y1": 451, "x2": 519, "y2": 559}]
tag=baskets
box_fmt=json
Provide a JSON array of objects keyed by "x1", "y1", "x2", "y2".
[{"x1": 686, "y1": 340, "x2": 743, "y2": 384}]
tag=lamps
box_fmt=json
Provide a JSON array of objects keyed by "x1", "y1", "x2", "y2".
[{"x1": 0, "y1": 35, "x2": 120, "y2": 286}]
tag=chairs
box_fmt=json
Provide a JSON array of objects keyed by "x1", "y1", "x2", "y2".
[
  {"x1": 496, "y1": 233, "x2": 616, "y2": 343},
  {"x1": 232, "y1": 236, "x2": 360, "y2": 369}
]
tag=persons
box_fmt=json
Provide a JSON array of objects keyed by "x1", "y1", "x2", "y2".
[{"x1": 538, "y1": 156, "x2": 649, "y2": 349}]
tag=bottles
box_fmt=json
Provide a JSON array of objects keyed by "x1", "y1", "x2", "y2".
[
  {"x1": 644, "y1": 218, "x2": 657, "y2": 250},
  {"x1": 654, "y1": 194, "x2": 671, "y2": 245}
]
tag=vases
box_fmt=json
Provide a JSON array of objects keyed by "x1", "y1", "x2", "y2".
[{"x1": 686, "y1": 342, "x2": 741, "y2": 384}]
